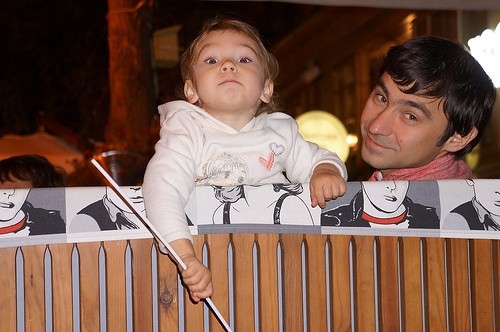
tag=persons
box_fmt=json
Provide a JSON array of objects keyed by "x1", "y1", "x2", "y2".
[
  {"x1": 142, "y1": 21, "x2": 348, "y2": 302},
  {"x1": 360, "y1": 36, "x2": 496, "y2": 181}
]
